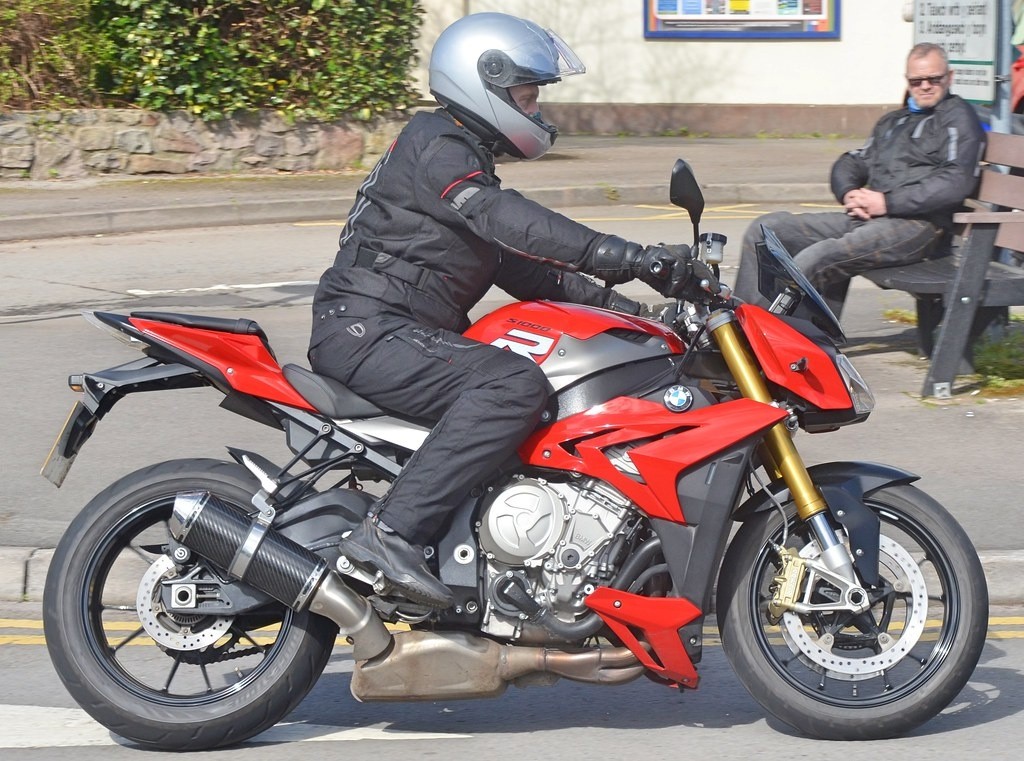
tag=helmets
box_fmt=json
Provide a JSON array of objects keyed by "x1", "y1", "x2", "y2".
[{"x1": 427, "y1": 11, "x2": 586, "y2": 163}]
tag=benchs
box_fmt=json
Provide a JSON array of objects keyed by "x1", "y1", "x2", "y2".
[{"x1": 857, "y1": 130, "x2": 1024, "y2": 399}]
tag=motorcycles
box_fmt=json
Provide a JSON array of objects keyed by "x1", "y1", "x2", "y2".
[{"x1": 35, "y1": 156, "x2": 992, "y2": 751}]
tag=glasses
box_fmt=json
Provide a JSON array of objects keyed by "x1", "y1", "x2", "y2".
[{"x1": 908, "y1": 71, "x2": 947, "y2": 86}]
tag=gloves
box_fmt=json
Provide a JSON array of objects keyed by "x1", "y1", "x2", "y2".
[
  {"x1": 637, "y1": 300, "x2": 680, "y2": 325},
  {"x1": 592, "y1": 231, "x2": 720, "y2": 303}
]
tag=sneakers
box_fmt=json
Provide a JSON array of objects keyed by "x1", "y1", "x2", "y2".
[{"x1": 340, "y1": 516, "x2": 455, "y2": 608}]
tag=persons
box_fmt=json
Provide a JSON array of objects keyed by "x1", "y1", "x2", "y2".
[
  {"x1": 305, "y1": 14, "x2": 723, "y2": 608},
  {"x1": 754, "y1": 42, "x2": 990, "y2": 312}
]
